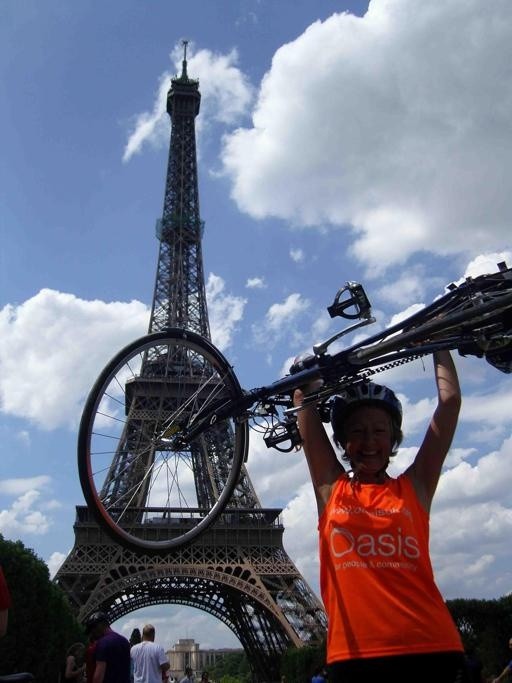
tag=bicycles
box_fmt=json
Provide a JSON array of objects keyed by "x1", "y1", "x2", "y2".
[{"x1": 77, "y1": 261, "x2": 512, "y2": 554}]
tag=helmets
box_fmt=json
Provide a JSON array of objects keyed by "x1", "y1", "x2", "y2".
[
  {"x1": 82, "y1": 611, "x2": 107, "y2": 626},
  {"x1": 330, "y1": 380, "x2": 402, "y2": 450}
]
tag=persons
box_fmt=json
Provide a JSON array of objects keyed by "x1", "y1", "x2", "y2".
[
  {"x1": 311, "y1": 665, "x2": 326, "y2": 683},
  {"x1": 293, "y1": 349, "x2": 465, "y2": 683},
  {"x1": 460, "y1": 649, "x2": 483, "y2": 683},
  {"x1": 493, "y1": 637, "x2": 512, "y2": 683},
  {"x1": 64, "y1": 611, "x2": 211, "y2": 683},
  {"x1": 0, "y1": 564, "x2": 12, "y2": 637}
]
